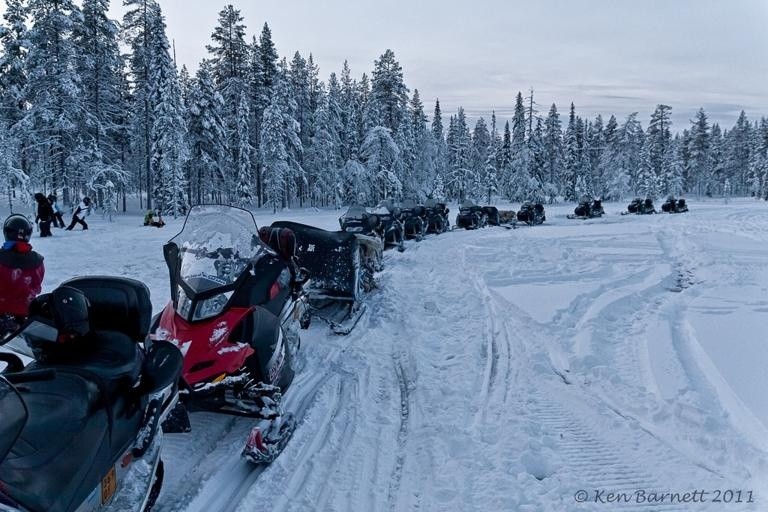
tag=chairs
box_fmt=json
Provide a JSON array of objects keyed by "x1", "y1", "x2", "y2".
[{"x1": 28, "y1": 275, "x2": 152, "y2": 447}]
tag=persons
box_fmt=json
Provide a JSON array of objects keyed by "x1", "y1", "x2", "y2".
[
  {"x1": 0, "y1": 213, "x2": 45, "y2": 344},
  {"x1": 34, "y1": 192, "x2": 54, "y2": 237},
  {"x1": 143, "y1": 207, "x2": 166, "y2": 227},
  {"x1": 47, "y1": 194, "x2": 65, "y2": 230},
  {"x1": 66, "y1": 197, "x2": 91, "y2": 232}
]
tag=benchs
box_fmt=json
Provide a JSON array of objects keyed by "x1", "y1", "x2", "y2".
[{"x1": 296, "y1": 227, "x2": 353, "y2": 294}]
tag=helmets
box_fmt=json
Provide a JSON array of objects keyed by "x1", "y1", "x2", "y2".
[{"x1": 3, "y1": 213, "x2": 33, "y2": 243}]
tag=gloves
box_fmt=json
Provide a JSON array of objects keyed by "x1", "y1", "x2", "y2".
[{"x1": 35, "y1": 216, "x2": 39, "y2": 223}]
